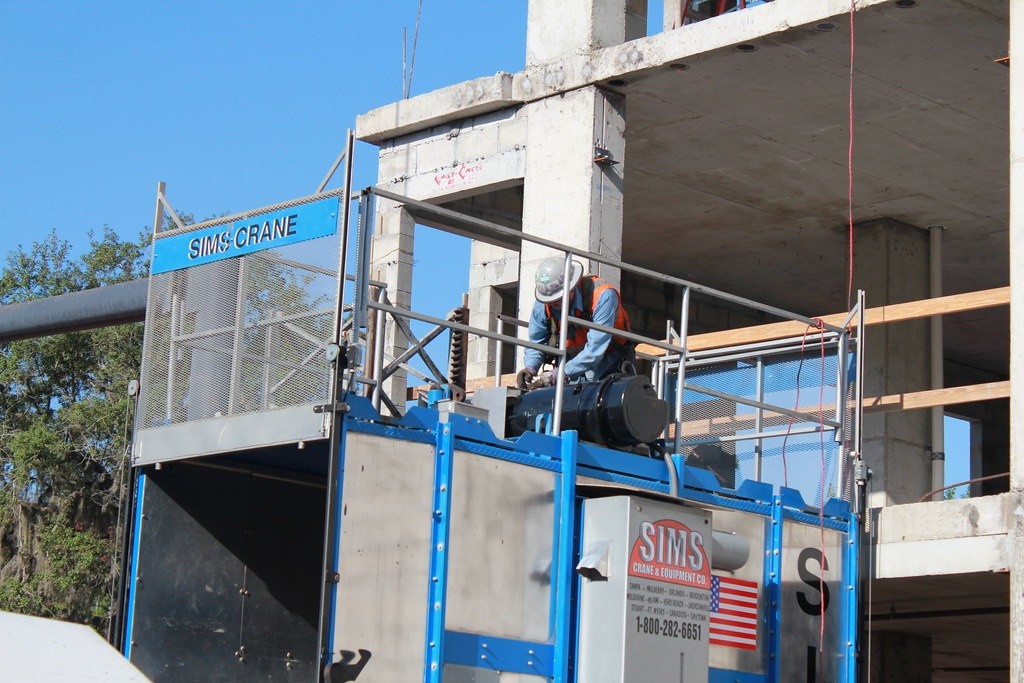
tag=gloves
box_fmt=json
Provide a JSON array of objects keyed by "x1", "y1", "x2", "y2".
[{"x1": 515, "y1": 366, "x2": 555, "y2": 393}]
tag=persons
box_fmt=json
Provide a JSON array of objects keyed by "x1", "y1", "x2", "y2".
[{"x1": 515, "y1": 258, "x2": 636, "y2": 393}]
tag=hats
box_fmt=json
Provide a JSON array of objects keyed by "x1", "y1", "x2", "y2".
[{"x1": 532, "y1": 255, "x2": 583, "y2": 302}]
